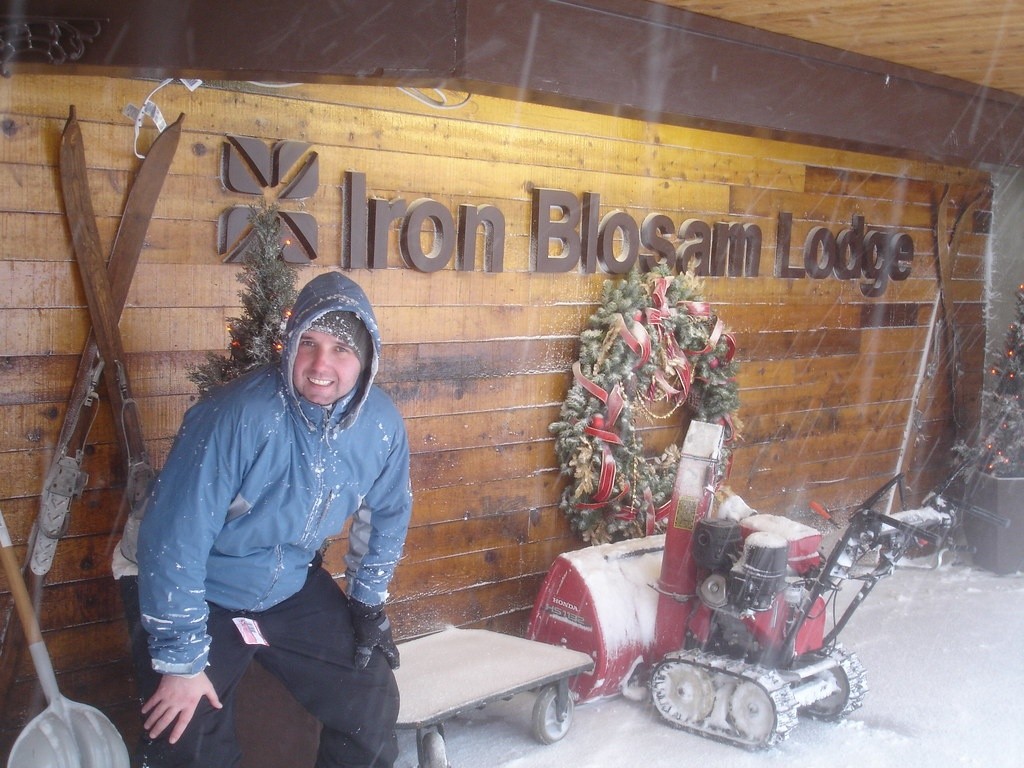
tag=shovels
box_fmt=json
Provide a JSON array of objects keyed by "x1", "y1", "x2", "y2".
[{"x1": 0, "y1": 502, "x2": 138, "y2": 767}]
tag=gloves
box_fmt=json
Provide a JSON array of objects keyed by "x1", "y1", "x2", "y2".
[{"x1": 349, "y1": 600, "x2": 401, "y2": 669}]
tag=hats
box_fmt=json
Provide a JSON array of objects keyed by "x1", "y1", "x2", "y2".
[{"x1": 311, "y1": 311, "x2": 368, "y2": 374}]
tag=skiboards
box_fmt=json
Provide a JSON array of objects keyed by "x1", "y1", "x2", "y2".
[
  {"x1": 929, "y1": 177, "x2": 992, "y2": 456},
  {"x1": 0, "y1": 94, "x2": 204, "y2": 738}
]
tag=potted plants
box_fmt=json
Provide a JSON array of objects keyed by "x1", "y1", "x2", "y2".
[{"x1": 946, "y1": 290, "x2": 1024, "y2": 577}]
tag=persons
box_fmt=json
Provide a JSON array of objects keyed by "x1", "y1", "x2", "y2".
[{"x1": 111, "y1": 271, "x2": 413, "y2": 768}]
